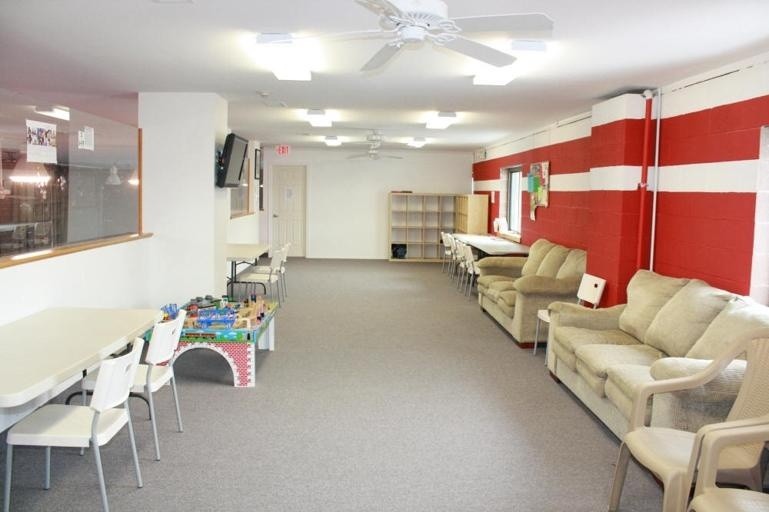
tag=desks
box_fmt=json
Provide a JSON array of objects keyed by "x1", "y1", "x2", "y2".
[
  {"x1": 146, "y1": 297, "x2": 281, "y2": 390},
  {"x1": 2, "y1": 302, "x2": 169, "y2": 511}
]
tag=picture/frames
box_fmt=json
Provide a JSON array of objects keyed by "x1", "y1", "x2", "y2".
[{"x1": 252, "y1": 148, "x2": 262, "y2": 181}]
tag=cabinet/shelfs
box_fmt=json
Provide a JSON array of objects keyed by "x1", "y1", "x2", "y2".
[{"x1": 386, "y1": 190, "x2": 490, "y2": 264}]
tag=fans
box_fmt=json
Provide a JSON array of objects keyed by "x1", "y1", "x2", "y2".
[
  {"x1": 303, "y1": 1, "x2": 556, "y2": 75},
  {"x1": 339, "y1": 133, "x2": 407, "y2": 164}
]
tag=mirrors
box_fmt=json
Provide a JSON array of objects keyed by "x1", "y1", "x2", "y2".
[{"x1": 0, "y1": 88, "x2": 157, "y2": 269}]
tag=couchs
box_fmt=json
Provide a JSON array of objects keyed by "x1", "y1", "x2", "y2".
[
  {"x1": 475, "y1": 239, "x2": 588, "y2": 348},
  {"x1": 543, "y1": 267, "x2": 769, "y2": 455}
]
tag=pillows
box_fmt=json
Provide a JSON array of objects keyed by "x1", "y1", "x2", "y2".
[
  {"x1": 682, "y1": 296, "x2": 768, "y2": 371},
  {"x1": 616, "y1": 269, "x2": 689, "y2": 344},
  {"x1": 520, "y1": 239, "x2": 586, "y2": 279},
  {"x1": 641, "y1": 277, "x2": 733, "y2": 358}
]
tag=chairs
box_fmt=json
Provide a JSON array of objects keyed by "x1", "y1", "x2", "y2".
[
  {"x1": 686, "y1": 423, "x2": 768, "y2": 512},
  {"x1": 226, "y1": 239, "x2": 294, "y2": 308},
  {"x1": 606, "y1": 325, "x2": 768, "y2": 512},
  {"x1": 531, "y1": 272, "x2": 607, "y2": 356},
  {"x1": 1, "y1": 335, "x2": 148, "y2": 512},
  {"x1": 78, "y1": 305, "x2": 189, "y2": 462},
  {"x1": 440, "y1": 229, "x2": 528, "y2": 301}
]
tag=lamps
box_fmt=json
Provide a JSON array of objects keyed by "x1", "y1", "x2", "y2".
[{"x1": 10, "y1": 149, "x2": 50, "y2": 184}]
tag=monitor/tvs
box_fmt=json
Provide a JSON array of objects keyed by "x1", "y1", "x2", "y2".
[{"x1": 216, "y1": 133, "x2": 249, "y2": 188}]
